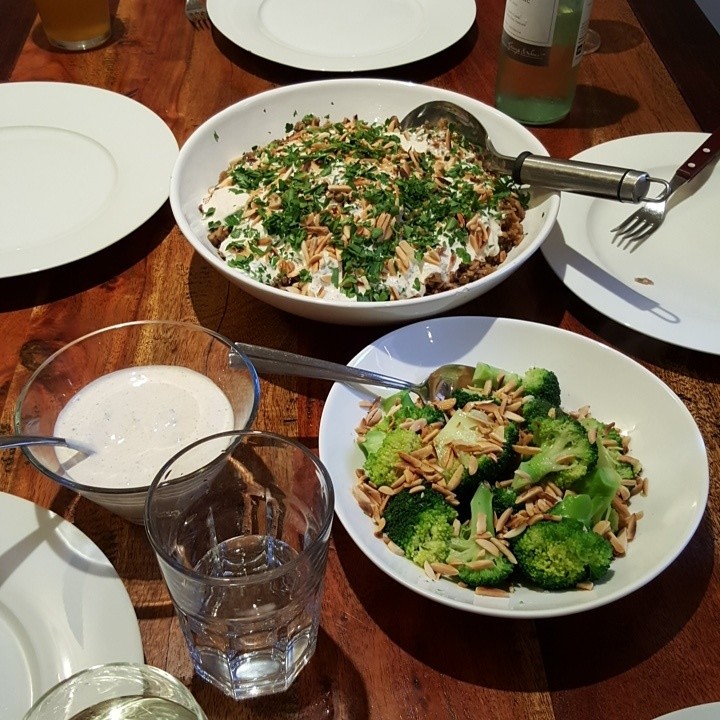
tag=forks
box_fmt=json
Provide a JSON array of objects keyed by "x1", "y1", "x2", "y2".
[
  {"x1": 185, "y1": 0, "x2": 212, "y2": 31},
  {"x1": 609, "y1": 129, "x2": 719, "y2": 240}
]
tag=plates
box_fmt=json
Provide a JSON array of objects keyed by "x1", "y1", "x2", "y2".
[
  {"x1": 0, "y1": 490, "x2": 144, "y2": 720},
  {"x1": 169, "y1": 77, "x2": 559, "y2": 325},
  {"x1": 316, "y1": 316, "x2": 710, "y2": 618},
  {"x1": 205, "y1": 0, "x2": 477, "y2": 72},
  {"x1": 540, "y1": 131, "x2": 720, "y2": 355},
  {"x1": 0, "y1": 80, "x2": 179, "y2": 279}
]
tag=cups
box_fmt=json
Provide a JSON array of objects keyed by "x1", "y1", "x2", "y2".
[
  {"x1": 143, "y1": 429, "x2": 334, "y2": 699},
  {"x1": 13, "y1": 320, "x2": 261, "y2": 526},
  {"x1": 23, "y1": 662, "x2": 207, "y2": 720},
  {"x1": 32, "y1": 0, "x2": 119, "y2": 51}
]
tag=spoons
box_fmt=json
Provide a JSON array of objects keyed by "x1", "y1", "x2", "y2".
[{"x1": 227, "y1": 336, "x2": 476, "y2": 407}]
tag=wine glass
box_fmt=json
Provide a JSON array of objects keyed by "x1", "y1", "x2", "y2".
[{"x1": 560, "y1": 1, "x2": 600, "y2": 54}]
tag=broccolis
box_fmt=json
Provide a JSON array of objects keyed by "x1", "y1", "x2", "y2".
[{"x1": 375, "y1": 361, "x2": 633, "y2": 608}]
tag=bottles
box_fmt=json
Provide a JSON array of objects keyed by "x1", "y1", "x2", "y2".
[{"x1": 495, "y1": 1, "x2": 593, "y2": 125}]
